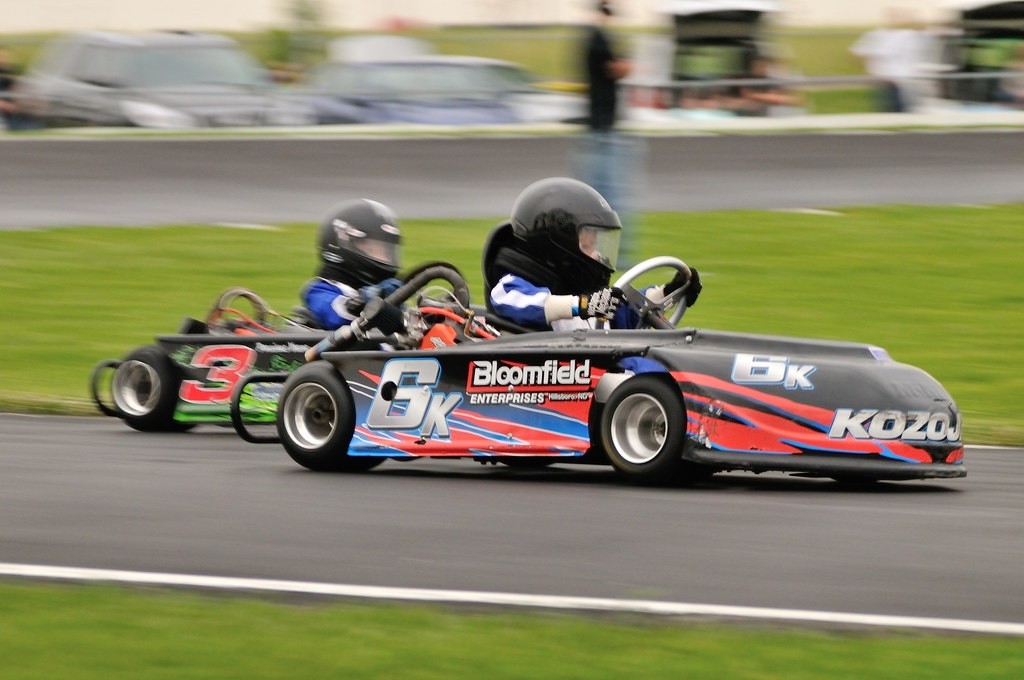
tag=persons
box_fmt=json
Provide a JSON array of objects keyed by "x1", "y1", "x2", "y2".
[
  {"x1": 490, "y1": 178, "x2": 702, "y2": 332},
  {"x1": 300, "y1": 198, "x2": 404, "y2": 331},
  {"x1": 0, "y1": 48, "x2": 48, "y2": 133},
  {"x1": 583, "y1": 0, "x2": 941, "y2": 135}
]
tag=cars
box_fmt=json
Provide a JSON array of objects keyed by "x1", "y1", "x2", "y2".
[
  {"x1": 2, "y1": 27, "x2": 322, "y2": 127},
  {"x1": 312, "y1": 54, "x2": 568, "y2": 126}
]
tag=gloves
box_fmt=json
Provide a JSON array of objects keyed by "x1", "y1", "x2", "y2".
[
  {"x1": 579, "y1": 287, "x2": 627, "y2": 319},
  {"x1": 664, "y1": 266, "x2": 701, "y2": 307},
  {"x1": 358, "y1": 280, "x2": 403, "y2": 300}
]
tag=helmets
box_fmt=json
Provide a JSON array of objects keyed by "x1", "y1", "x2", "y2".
[
  {"x1": 316, "y1": 198, "x2": 407, "y2": 287},
  {"x1": 511, "y1": 177, "x2": 621, "y2": 290}
]
